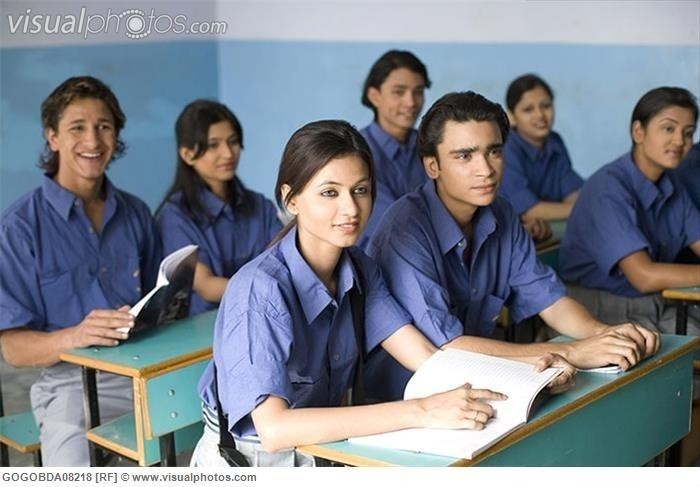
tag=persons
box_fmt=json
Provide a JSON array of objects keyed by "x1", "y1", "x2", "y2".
[
  {"x1": 559, "y1": 83, "x2": 699, "y2": 339},
  {"x1": 153, "y1": 96, "x2": 286, "y2": 319},
  {"x1": 0, "y1": 74, "x2": 165, "y2": 466},
  {"x1": 677, "y1": 142, "x2": 699, "y2": 211},
  {"x1": 359, "y1": 90, "x2": 661, "y2": 373},
  {"x1": 499, "y1": 73, "x2": 588, "y2": 224},
  {"x1": 353, "y1": 47, "x2": 552, "y2": 251},
  {"x1": 185, "y1": 117, "x2": 578, "y2": 467}
]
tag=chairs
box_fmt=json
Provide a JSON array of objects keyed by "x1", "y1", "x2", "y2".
[
  {"x1": 142, "y1": 353, "x2": 224, "y2": 466},
  {"x1": 1, "y1": 377, "x2": 49, "y2": 465}
]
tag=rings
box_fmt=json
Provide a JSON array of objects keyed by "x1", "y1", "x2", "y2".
[{"x1": 470, "y1": 409, "x2": 480, "y2": 420}]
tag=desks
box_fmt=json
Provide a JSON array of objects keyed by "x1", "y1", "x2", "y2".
[
  {"x1": 295, "y1": 328, "x2": 699, "y2": 465},
  {"x1": 662, "y1": 277, "x2": 699, "y2": 333},
  {"x1": 58, "y1": 301, "x2": 225, "y2": 466}
]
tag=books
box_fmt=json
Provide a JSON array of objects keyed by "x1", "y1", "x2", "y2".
[
  {"x1": 570, "y1": 363, "x2": 624, "y2": 374},
  {"x1": 344, "y1": 345, "x2": 569, "y2": 461},
  {"x1": 108, "y1": 243, "x2": 199, "y2": 337}
]
